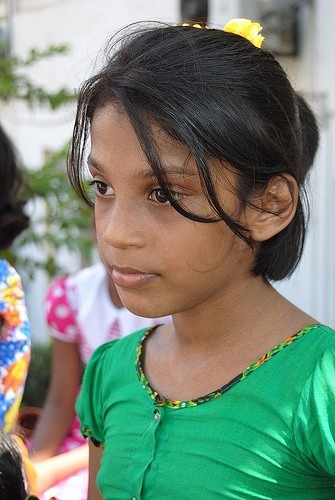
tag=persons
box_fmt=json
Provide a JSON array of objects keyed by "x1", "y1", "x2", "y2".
[
  {"x1": 0, "y1": 124, "x2": 173, "y2": 500},
  {"x1": 66, "y1": 20, "x2": 335, "y2": 500}
]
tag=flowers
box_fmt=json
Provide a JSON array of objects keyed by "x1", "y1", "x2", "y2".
[{"x1": 170, "y1": 17, "x2": 265, "y2": 50}]
